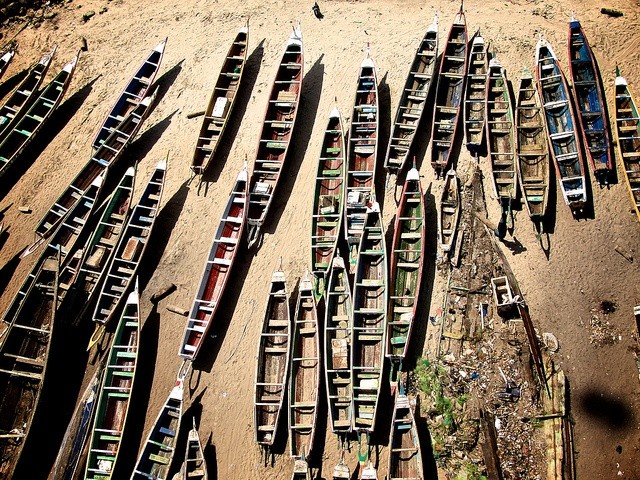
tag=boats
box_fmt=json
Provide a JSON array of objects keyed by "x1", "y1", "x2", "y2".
[
  {"x1": 382, "y1": 383, "x2": 424, "y2": 479},
  {"x1": 129, "y1": 376, "x2": 183, "y2": 480},
  {"x1": 83, "y1": 275, "x2": 141, "y2": 480},
  {"x1": 3, "y1": 166, "x2": 107, "y2": 327},
  {"x1": 83, "y1": 150, "x2": 170, "y2": 352},
  {"x1": 323, "y1": 249, "x2": 353, "y2": 449},
  {"x1": 72, "y1": 158, "x2": 139, "y2": 334},
  {"x1": 437, "y1": 161, "x2": 459, "y2": 252},
  {"x1": 0, "y1": 43, "x2": 18, "y2": 74},
  {"x1": 0, "y1": 250, "x2": 61, "y2": 479},
  {"x1": 535, "y1": 33, "x2": 588, "y2": 217},
  {"x1": 568, "y1": 20, "x2": 617, "y2": 189},
  {"x1": 89, "y1": 36, "x2": 167, "y2": 152},
  {"x1": 253, "y1": 258, "x2": 292, "y2": 461},
  {"x1": 56, "y1": 249, "x2": 81, "y2": 311},
  {"x1": 464, "y1": 25, "x2": 488, "y2": 146},
  {"x1": 0, "y1": 44, "x2": 59, "y2": 138},
  {"x1": 333, "y1": 464, "x2": 351, "y2": 480},
  {"x1": 178, "y1": 153, "x2": 247, "y2": 362},
  {"x1": 429, "y1": 7, "x2": 468, "y2": 176},
  {"x1": 485, "y1": 45, "x2": 518, "y2": 230},
  {"x1": 312, "y1": 93, "x2": 347, "y2": 298},
  {"x1": 386, "y1": 154, "x2": 428, "y2": 392},
  {"x1": 186, "y1": 15, "x2": 249, "y2": 188},
  {"x1": 246, "y1": 20, "x2": 303, "y2": 248},
  {"x1": 288, "y1": 271, "x2": 322, "y2": 480},
  {"x1": 0, "y1": 49, "x2": 81, "y2": 178},
  {"x1": 183, "y1": 416, "x2": 208, "y2": 480},
  {"x1": 614, "y1": 64, "x2": 640, "y2": 221},
  {"x1": 28, "y1": 84, "x2": 159, "y2": 238},
  {"x1": 382, "y1": 11, "x2": 439, "y2": 174},
  {"x1": 347, "y1": 59, "x2": 379, "y2": 267},
  {"x1": 358, "y1": 464, "x2": 378, "y2": 480},
  {"x1": 352, "y1": 200, "x2": 387, "y2": 470},
  {"x1": 515, "y1": 66, "x2": 550, "y2": 243},
  {"x1": 290, "y1": 459, "x2": 309, "y2": 480}
]
